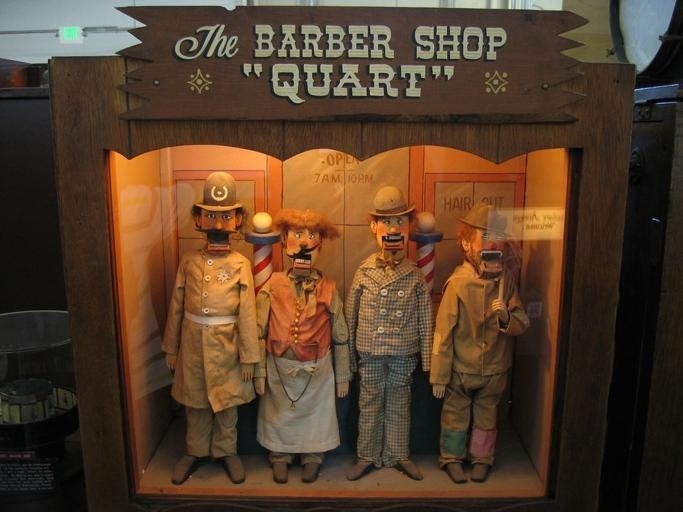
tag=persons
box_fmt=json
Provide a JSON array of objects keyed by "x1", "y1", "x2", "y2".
[
  {"x1": 249, "y1": 205, "x2": 352, "y2": 485},
  {"x1": 156, "y1": 170, "x2": 262, "y2": 486},
  {"x1": 426, "y1": 201, "x2": 531, "y2": 484},
  {"x1": 339, "y1": 186, "x2": 434, "y2": 484}
]
